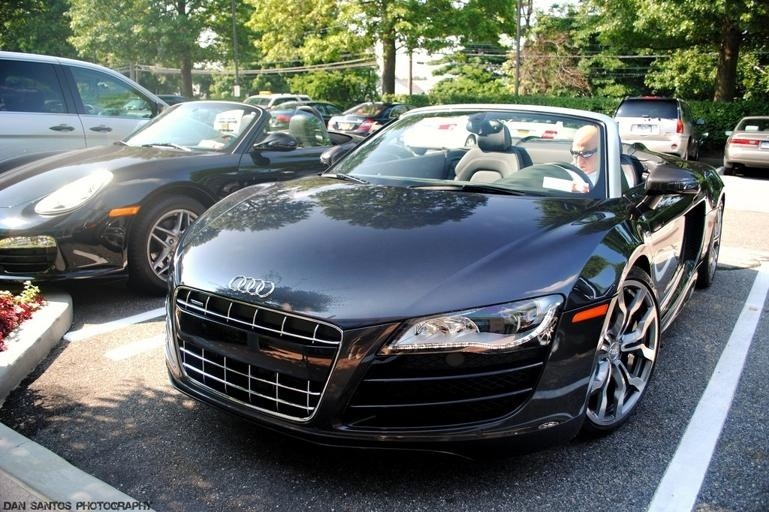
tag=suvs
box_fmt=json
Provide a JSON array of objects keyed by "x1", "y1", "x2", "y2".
[{"x1": 611, "y1": 96, "x2": 705, "y2": 160}]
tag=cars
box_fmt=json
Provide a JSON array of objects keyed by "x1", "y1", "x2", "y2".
[{"x1": 723, "y1": 115, "x2": 769, "y2": 175}]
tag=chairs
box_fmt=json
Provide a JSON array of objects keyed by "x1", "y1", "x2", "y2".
[
  {"x1": 745, "y1": 125, "x2": 759, "y2": 131},
  {"x1": 289, "y1": 106, "x2": 328, "y2": 148},
  {"x1": 449, "y1": 126, "x2": 524, "y2": 184},
  {"x1": 238, "y1": 114, "x2": 257, "y2": 137}
]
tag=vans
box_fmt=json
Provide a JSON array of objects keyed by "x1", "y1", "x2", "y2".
[{"x1": 1, "y1": 49, "x2": 169, "y2": 180}]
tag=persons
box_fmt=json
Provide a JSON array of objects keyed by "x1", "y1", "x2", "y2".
[{"x1": 564, "y1": 125, "x2": 599, "y2": 194}]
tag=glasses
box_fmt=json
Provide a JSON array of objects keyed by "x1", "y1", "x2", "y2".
[{"x1": 571, "y1": 147, "x2": 597, "y2": 158}]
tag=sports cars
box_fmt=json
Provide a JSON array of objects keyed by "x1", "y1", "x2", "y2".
[
  {"x1": 166, "y1": 101, "x2": 724, "y2": 465},
  {"x1": 1, "y1": 99, "x2": 372, "y2": 292}
]
